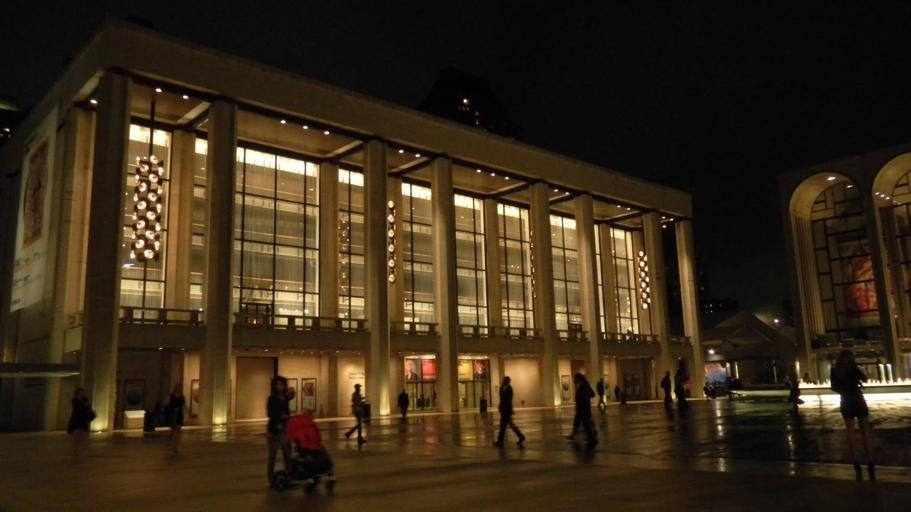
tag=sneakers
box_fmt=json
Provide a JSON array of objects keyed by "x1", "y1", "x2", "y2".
[
  {"x1": 853, "y1": 463, "x2": 862, "y2": 472},
  {"x1": 344, "y1": 431, "x2": 352, "y2": 439},
  {"x1": 516, "y1": 435, "x2": 526, "y2": 445},
  {"x1": 492, "y1": 441, "x2": 504, "y2": 448},
  {"x1": 867, "y1": 455, "x2": 876, "y2": 470},
  {"x1": 357, "y1": 437, "x2": 367, "y2": 445}
]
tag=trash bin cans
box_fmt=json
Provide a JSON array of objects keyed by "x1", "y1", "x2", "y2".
[
  {"x1": 362, "y1": 400, "x2": 371, "y2": 423},
  {"x1": 144, "y1": 408, "x2": 155, "y2": 432},
  {"x1": 620, "y1": 390, "x2": 627, "y2": 404},
  {"x1": 480, "y1": 399, "x2": 487, "y2": 413}
]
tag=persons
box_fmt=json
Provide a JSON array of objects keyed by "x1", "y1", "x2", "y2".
[
  {"x1": 491, "y1": 377, "x2": 526, "y2": 449},
  {"x1": 345, "y1": 384, "x2": 367, "y2": 446},
  {"x1": 614, "y1": 384, "x2": 621, "y2": 400},
  {"x1": 782, "y1": 362, "x2": 811, "y2": 403},
  {"x1": 67, "y1": 386, "x2": 96, "y2": 465},
  {"x1": 700, "y1": 374, "x2": 753, "y2": 401},
  {"x1": 674, "y1": 370, "x2": 688, "y2": 407},
  {"x1": 830, "y1": 350, "x2": 876, "y2": 473},
  {"x1": 264, "y1": 375, "x2": 297, "y2": 482},
  {"x1": 165, "y1": 380, "x2": 193, "y2": 456},
  {"x1": 396, "y1": 388, "x2": 409, "y2": 418},
  {"x1": 563, "y1": 373, "x2": 599, "y2": 445},
  {"x1": 598, "y1": 380, "x2": 610, "y2": 409},
  {"x1": 662, "y1": 370, "x2": 674, "y2": 407}
]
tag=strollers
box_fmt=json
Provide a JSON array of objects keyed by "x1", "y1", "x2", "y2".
[{"x1": 269, "y1": 412, "x2": 337, "y2": 496}]
